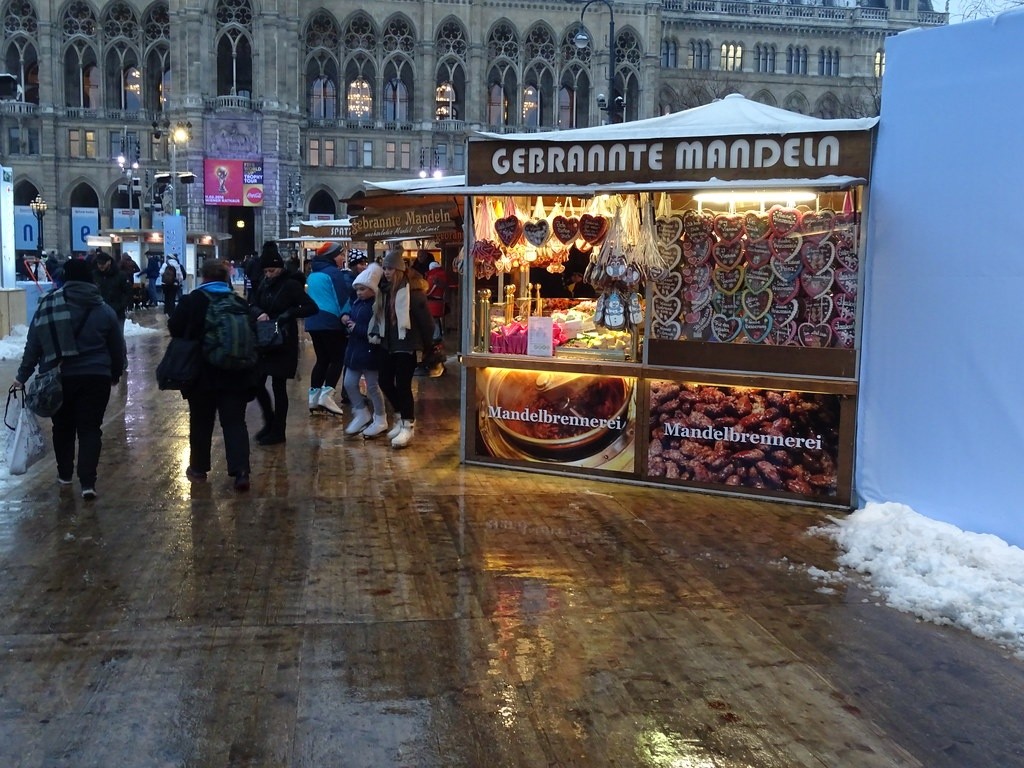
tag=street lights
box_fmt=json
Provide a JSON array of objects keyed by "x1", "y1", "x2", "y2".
[
  {"x1": 170, "y1": 125, "x2": 187, "y2": 215},
  {"x1": 116, "y1": 155, "x2": 139, "y2": 231},
  {"x1": 29, "y1": 194, "x2": 48, "y2": 280},
  {"x1": 573, "y1": 0, "x2": 627, "y2": 124}
]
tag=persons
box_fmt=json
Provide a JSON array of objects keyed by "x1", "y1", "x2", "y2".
[
  {"x1": 86, "y1": 247, "x2": 141, "y2": 340},
  {"x1": 14, "y1": 259, "x2": 128, "y2": 500},
  {"x1": 159, "y1": 253, "x2": 187, "y2": 316},
  {"x1": 249, "y1": 240, "x2": 323, "y2": 447},
  {"x1": 224, "y1": 251, "x2": 262, "y2": 296},
  {"x1": 15, "y1": 251, "x2": 83, "y2": 282},
  {"x1": 139, "y1": 250, "x2": 159, "y2": 306},
  {"x1": 303, "y1": 241, "x2": 448, "y2": 448},
  {"x1": 167, "y1": 258, "x2": 271, "y2": 493},
  {"x1": 287, "y1": 249, "x2": 302, "y2": 272}
]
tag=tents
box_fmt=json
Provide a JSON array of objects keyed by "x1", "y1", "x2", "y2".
[
  {"x1": 338, "y1": 173, "x2": 469, "y2": 263},
  {"x1": 454, "y1": 90, "x2": 881, "y2": 509}
]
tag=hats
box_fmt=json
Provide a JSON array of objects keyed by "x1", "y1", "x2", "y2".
[
  {"x1": 259, "y1": 241, "x2": 283, "y2": 269},
  {"x1": 382, "y1": 244, "x2": 406, "y2": 271},
  {"x1": 96, "y1": 252, "x2": 110, "y2": 264},
  {"x1": 352, "y1": 263, "x2": 383, "y2": 290},
  {"x1": 317, "y1": 242, "x2": 344, "y2": 258}
]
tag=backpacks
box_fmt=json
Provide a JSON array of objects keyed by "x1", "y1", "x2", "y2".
[
  {"x1": 199, "y1": 289, "x2": 257, "y2": 369},
  {"x1": 162, "y1": 260, "x2": 176, "y2": 283}
]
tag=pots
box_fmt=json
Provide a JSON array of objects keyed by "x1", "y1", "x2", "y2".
[{"x1": 487, "y1": 368, "x2": 634, "y2": 456}]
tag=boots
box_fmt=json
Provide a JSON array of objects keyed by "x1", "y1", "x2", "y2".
[
  {"x1": 256, "y1": 411, "x2": 287, "y2": 446},
  {"x1": 344, "y1": 406, "x2": 372, "y2": 434},
  {"x1": 363, "y1": 412, "x2": 389, "y2": 436},
  {"x1": 308, "y1": 388, "x2": 324, "y2": 411},
  {"x1": 317, "y1": 385, "x2": 343, "y2": 414},
  {"x1": 387, "y1": 413, "x2": 403, "y2": 437},
  {"x1": 390, "y1": 418, "x2": 416, "y2": 445}
]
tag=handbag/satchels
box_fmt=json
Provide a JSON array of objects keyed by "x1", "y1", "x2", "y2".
[
  {"x1": 255, "y1": 318, "x2": 282, "y2": 346},
  {"x1": 25, "y1": 366, "x2": 64, "y2": 416},
  {"x1": 8, "y1": 385, "x2": 48, "y2": 475}
]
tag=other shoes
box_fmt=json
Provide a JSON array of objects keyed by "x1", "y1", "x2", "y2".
[
  {"x1": 414, "y1": 364, "x2": 430, "y2": 375},
  {"x1": 234, "y1": 477, "x2": 251, "y2": 491},
  {"x1": 57, "y1": 471, "x2": 71, "y2": 482},
  {"x1": 428, "y1": 362, "x2": 445, "y2": 377},
  {"x1": 188, "y1": 465, "x2": 208, "y2": 478},
  {"x1": 82, "y1": 484, "x2": 98, "y2": 498}
]
